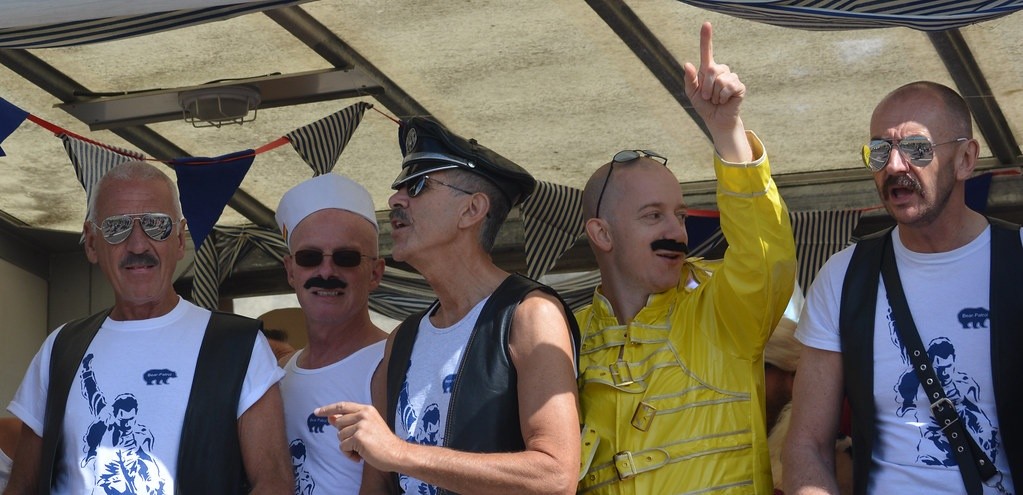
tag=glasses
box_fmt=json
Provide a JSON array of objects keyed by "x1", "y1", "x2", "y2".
[
  {"x1": 87, "y1": 212, "x2": 179, "y2": 244},
  {"x1": 595, "y1": 149, "x2": 668, "y2": 220},
  {"x1": 860, "y1": 134, "x2": 968, "y2": 172},
  {"x1": 397, "y1": 174, "x2": 491, "y2": 219},
  {"x1": 289, "y1": 246, "x2": 375, "y2": 268}
]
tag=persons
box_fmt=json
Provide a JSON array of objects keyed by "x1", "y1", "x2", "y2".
[
  {"x1": 752, "y1": 316, "x2": 852, "y2": 495},
  {"x1": 313, "y1": 115, "x2": 580, "y2": 495},
  {"x1": 570, "y1": 22, "x2": 797, "y2": 495},
  {"x1": 273, "y1": 173, "x2": 392, "y2": 495},
  {"x1": 256, "y1": 329, "x2": 294, "y2": 367},
  {"x1": 3, "y1": 161, "x2": 298, "y2": 495},
  {"x1": 781, "y1": 82, "x2": 1023, "y2": 495}
]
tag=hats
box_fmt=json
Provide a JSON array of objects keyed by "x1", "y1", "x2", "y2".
[
  {"x1": 389, "y1": 115, "x2": 538, "y2": 209},
  {"x1": 274, "y1": 173, "x2": 379, "y2": 247}
]
